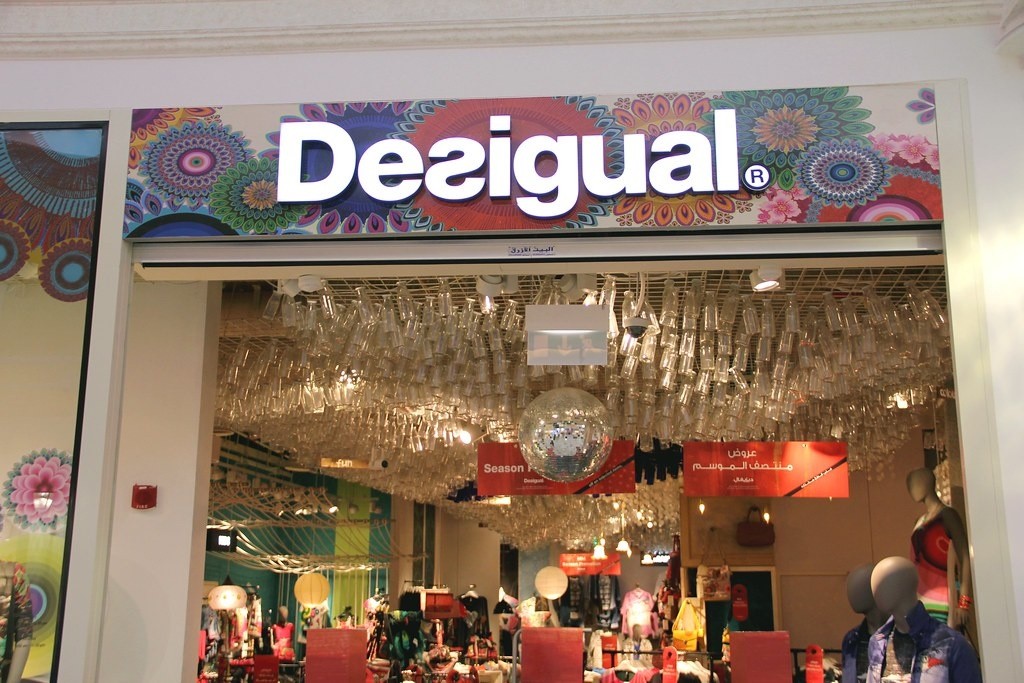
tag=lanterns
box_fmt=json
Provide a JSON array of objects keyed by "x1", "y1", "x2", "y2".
[{"x1": 295, "y1": 573, "x2": 331, "y2": 608}]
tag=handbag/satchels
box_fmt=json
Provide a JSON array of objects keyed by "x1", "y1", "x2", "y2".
[
  {"x1": 696, "y1": 527, "x2": 732, "y2": 602},
  {"x1": 736, "y1": 506, "x2": 775, "y2": 547},
  {"x1": 672, "y1": 599, "x2": 706, "y2": 653},
  {"x1": 666, "y1": 535, "x2": 680, "y2": 581}
]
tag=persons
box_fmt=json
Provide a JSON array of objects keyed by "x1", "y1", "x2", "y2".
[
  {"x1": 842, "y1": 567, "x2": 891, "y2": 683},
  {"x1": 334, "y1": 606, "x2": 358, "y2": 629},
  {"x1": 365, "y1": 588, "x2": 389, "y2": 636},
  {"x1": 907, "y1": 467, "x2": 973, "y2": 635},
  {"x1": 0, "y1": 560, "x2": 33, "y2": 683},
  {"x1": 623, "y1": 624, "x2": 653, "y2": 664},
  {"x1": 271, "y1": 606, "x2": 294, "y2": 664},
  {"x1": 866, "y1": 557, "x2": 982, "y2": 683}
]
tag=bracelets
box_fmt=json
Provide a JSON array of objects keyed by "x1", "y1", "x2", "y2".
[{"x1": 957, "y1": 595, "x2": 973, "y2": 611}]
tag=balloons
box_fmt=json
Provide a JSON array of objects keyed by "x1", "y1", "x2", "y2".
[{"x1": 534, "y1": 565, "x2": 568, "y2": 601}]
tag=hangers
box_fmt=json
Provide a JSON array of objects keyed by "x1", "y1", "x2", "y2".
[
  {"x1": 614, "y1": 650, "x2": 706, "y2": 674},
  {"x1": 367, "y1": 584, "x2": 479, "y2": 607}
]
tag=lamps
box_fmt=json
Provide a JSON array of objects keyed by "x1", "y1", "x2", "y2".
[
  {"x1": 210, "y1": 463, "x2": 386, "y2": 516},
  {"x1": 591, "y1": 540, "x2": 608, "y2": 560},
  {"x1": 208, "y1": 552, "x2": 247, "y2": 610},
  {"x1": 763, "y1": 506, "x2": 770, "y2": 524},
  {"x1": 616, "y1": 513, "x2": 629, "y2": 552},
  {"x1": 613, "y1": 501, "x2": 621, "y2": 511},
  {"x1": 749, "y1": 269, "x2": 779, "y2": 292},
  {"x1": 698, "y1": 498, "x2": 705, "y2": 514},
  {"x1": 460, "y1": 422, "x2": 489, "y2": 444},
  {"x1": 551, "y1": 274, "x2": 585, "y2": 303},
  {"x1": 293, "y1": 515, "x2": 330, "y2": 606},
  {"x1": 281, "y1": 279, "x2": 300, "y2": 297},
  {"x1": 475, "y1": 275, "x2": 503, "y2": 297}
]
